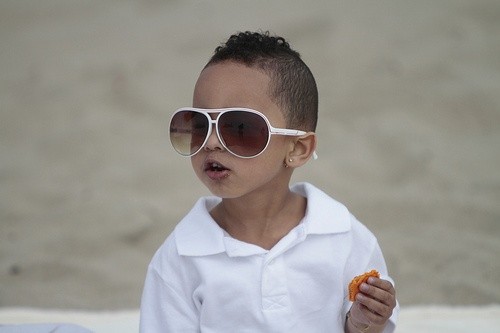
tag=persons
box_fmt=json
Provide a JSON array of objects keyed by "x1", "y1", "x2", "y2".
[{"x1": 139, "y1": 31, "x2": 403, "y2": 333}]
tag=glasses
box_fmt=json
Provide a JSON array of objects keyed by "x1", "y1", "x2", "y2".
[{"x1": 167, "y1": 107, "x2": 318, "y2": 162}]
